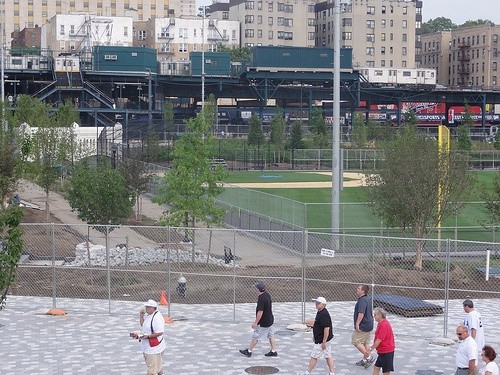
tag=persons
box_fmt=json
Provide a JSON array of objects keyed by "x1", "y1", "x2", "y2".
[
  {"x1": 305, "y1": 297, "x2": 334, "y2": 375},
  {"x1": 455, "y1": 325, "x2": 479, "y2": 375},
  {"x1": 351, "y1": 285, "x2": 376, "y2": 369},
  {"x1": 368, "y1": 307, "x2": 395, "y2": 375},
  {"x1": 481, "y1": 346, "x2": 499, "y2": 375},
  {"x1": 240, "y1": 284, "x2": 277, "y2": 357},
  {"x1": 462, "y1": 300, "x2": 485, "y2": 375},
  {"x1": 140, "y1": 300, "x2": 166, "y2": 375}
]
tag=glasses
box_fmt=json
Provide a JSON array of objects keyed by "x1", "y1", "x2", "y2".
[{"x1": 457, "y1": 331, "x2": 465, "y2": 335}]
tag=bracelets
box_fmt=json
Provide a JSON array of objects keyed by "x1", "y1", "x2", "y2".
[
  {"x1": 323, "y1": 340, "x2": 326, "y2": 343},
  {"x1": 369, "y1": 346, "x2": 373, "y2": 351},
  {"x1": 148, "y1": 335, "x2": 150, "y2": 339}
]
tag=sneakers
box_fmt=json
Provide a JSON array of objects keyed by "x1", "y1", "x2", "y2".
[
  {"x1": 265, "y1": 351, "x2": 277, "y2": 356},
  {"x1": 239, "y1": 349, "x2": 252, "y2": 357},
  {"x1": 356, "y1": 359, "x2": 367, "y2": 366},
  {"x1": 364, "y1": 356, "x2": 376, "y2": 368}
]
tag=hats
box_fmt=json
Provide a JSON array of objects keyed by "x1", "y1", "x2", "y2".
[
  {"x1": 255, "y1": 283, "x2": 266, "y2": 291},
  {"x1": 312, "y1": 297, "x2": 326, "y2": 304},
  {"x1": 144, "y1": 299, "x2": 158, "y2": 308}
]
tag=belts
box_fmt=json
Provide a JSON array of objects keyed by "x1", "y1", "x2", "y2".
[{"x1": 458, "y1": 367, "x2": 469, "y2": 369}]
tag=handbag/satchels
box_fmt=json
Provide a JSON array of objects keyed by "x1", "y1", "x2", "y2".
[{"x1": 149, "y1": 333, "x2": 160, "y2": 347}]
